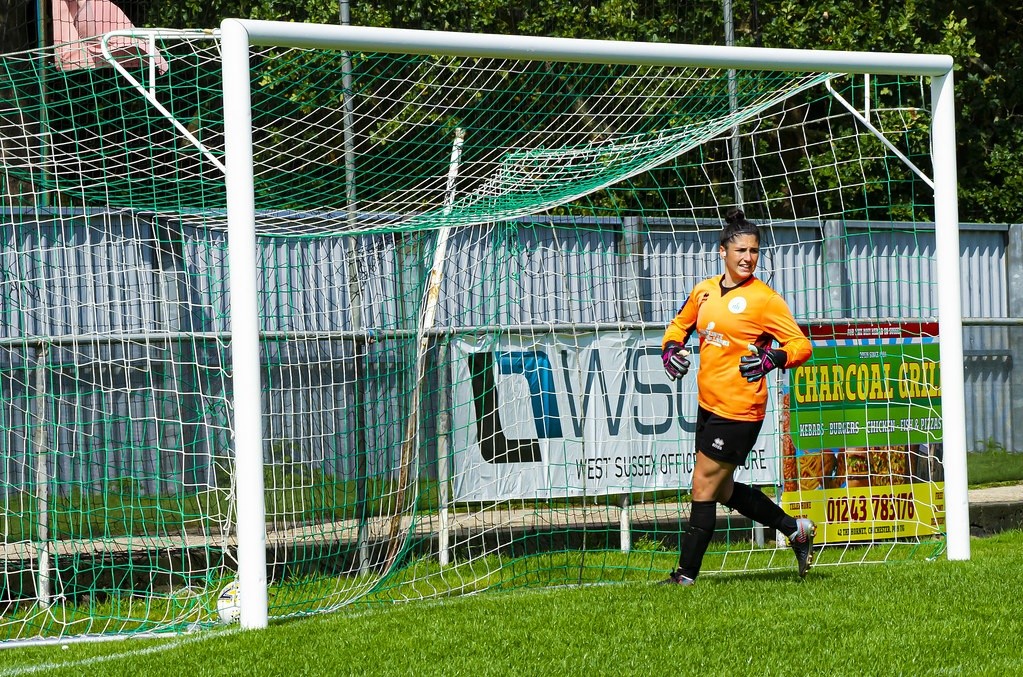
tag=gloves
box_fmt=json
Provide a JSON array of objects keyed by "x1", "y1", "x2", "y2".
[
  {"x1": 740, "y1": 344, "x2": 786, "y2": 382},
  {"x1": 661, "y1": 342, "x2": 692, "y2": 381}
]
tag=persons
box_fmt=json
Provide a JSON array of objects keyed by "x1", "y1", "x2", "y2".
[{"x1": 659, "y1": 209, "x2": 817, "y2": 587}]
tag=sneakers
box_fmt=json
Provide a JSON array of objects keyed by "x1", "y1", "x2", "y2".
[
  {"x1": 656, "y1": 567, "x2": 695, "y2": 586},
  {"x1": 788, "y1": 518, "x2": 818, "y2": 577}
]
